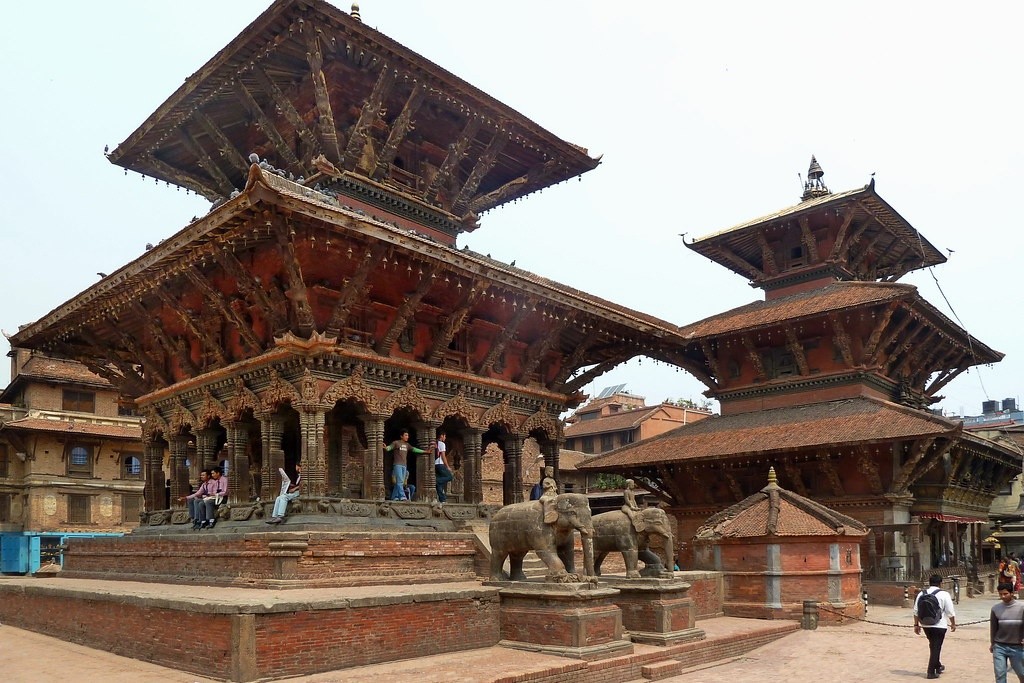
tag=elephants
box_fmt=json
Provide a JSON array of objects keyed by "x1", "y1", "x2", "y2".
[
  {"x1": 489, "y1": 491, "x2": 599, "y2": 583},
  {"x1": 582, "y1": 507, "x2": 675, "y2": 579}
]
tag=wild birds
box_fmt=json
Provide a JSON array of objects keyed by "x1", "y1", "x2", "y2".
[{"x1": 97, "y1": 148, "x2": 516, "y2": 278}]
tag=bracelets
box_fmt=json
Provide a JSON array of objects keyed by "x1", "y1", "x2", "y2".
[{"x1": 914, "y1": 624, "x2": 918, "y2": 626}]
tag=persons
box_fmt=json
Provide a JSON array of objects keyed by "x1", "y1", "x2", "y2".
[
  {"x1": 434, "y1": 432, "x2": 455, "y2": 503},
  {"x1": 264, "y1": 460, "x2": 300, "y2": 524},
  {"x1": 674, "y1": 558, "x2": 680, "y2": 571},
  {"x1": 621, "y1": 478, "x2": 640, "y2": 520},
  {"x1": 179, "y1": 466, "x2": 228, "y2": 529},
  {"x1": 989, "y1": 582, "x2": 1024, "y2": 683},
  {"x1": 914, "y1": 574, "x2": 956, "y2": 680},
  {"x1": 540, "y1": 466, "x2": 558, "y2": 523},
  {"x1": 998, "y1": 552, "x2": 1024, "y2": 591},
  {"x1": 383, "y1": 428, "x2": 433, "y2": 501}
]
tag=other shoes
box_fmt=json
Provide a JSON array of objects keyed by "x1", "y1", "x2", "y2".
[
  {"x1": 936, "y1": 666, "x2": 944, "y2": 673},
  {"x1": 273, "y1": 517, "x2": 285, "y2": 523},
  {"x1": 199, "y1": 520, "x2": 208, "y2": 529},
  {"x1": 265, "y1": 519, "x2": 275, "y2": 524},
  {"x1": 205, "y1": 519, "x2": 216, "y2": 529},
  {"x1": 400, "y1": 497, "x2": 406, "y2": 501},
  {"x1": 192, "y1": 521, "x2": 200, "y2": 529},
  {"x1": 393, "y1": 497, "x2": 399, "y2": 501},
  {"x1": 927, "y1": 673, "x2": 939, "y2": 679}
]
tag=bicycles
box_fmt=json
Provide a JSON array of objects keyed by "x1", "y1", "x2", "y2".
[{"x1": 948, "y1": 574, "x2": 961, "y2": 604}]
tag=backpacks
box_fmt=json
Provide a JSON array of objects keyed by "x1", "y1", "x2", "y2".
[{"x1": 918, "y1": 589, "x2": 943, "y2": 625}]
